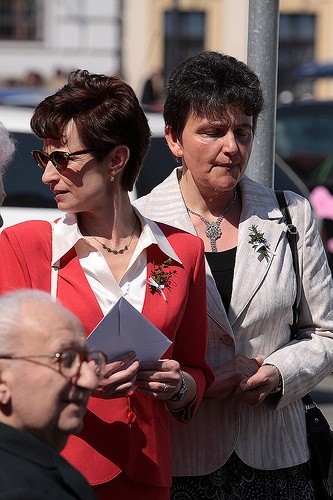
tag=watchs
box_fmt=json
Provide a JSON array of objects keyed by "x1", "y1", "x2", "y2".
[
  {"x1": 268, "y1": 365, "x2": 283, "y2": 395},
  {"x1": 168, "y1": 370, "x2": 188, "y2": 402}
]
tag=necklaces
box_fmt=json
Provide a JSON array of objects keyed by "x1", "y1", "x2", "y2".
[
  {"x1": 78, "y1": 215, "x2": 137, "y2": 255},
  {"x1": 186, "y1": 187, "x2": 237, "y2": 252}
]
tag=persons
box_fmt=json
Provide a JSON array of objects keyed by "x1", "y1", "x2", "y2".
[{"x1": 0, "y1": 51, "x2": 333, "y2": 500}]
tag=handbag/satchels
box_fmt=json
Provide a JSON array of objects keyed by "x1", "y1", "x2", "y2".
[{"x1": 305, "y1": 407, "x2": 333, "y2": 482}]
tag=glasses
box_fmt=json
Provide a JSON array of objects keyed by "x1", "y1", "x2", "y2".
[
  {"x1": 0, "y1": 348, "x2": 107, "y2": 379},
  {"x1": 31, "y1": 146, "x2": 113, "y2": 171}
]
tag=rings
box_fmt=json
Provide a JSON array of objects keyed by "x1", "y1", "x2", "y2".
[{"x1": 163, "y1": 383, "x2": 169, "y2": 392}]
